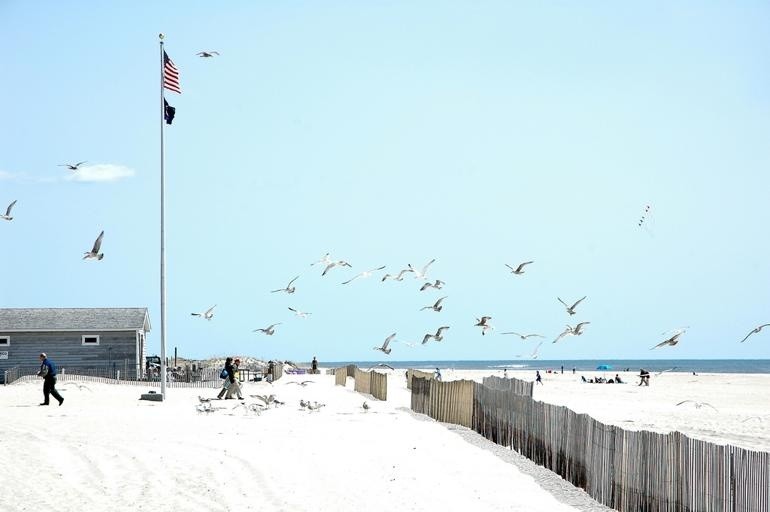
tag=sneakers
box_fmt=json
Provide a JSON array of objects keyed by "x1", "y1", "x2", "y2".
[
  {"x1": 238, "y1": 397, "x2": 245, "y2": 400},
  {"x1": 217, "y1": 395, "x2": 222, "y2": 400},
  {"x1": 40, "y1": 403, "x2": 49, "y2": 406},
  {"x1": 225, "y1": 396, "x2": 236, "y2": 399},
  {"x1": 58, "y1": 398, "x2": 64, "y2": 406}
]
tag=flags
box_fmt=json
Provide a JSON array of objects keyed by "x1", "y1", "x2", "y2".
[
  {"x1": 163, "y1": 51, "x2": 181, "y2": 95},
  {"x1": 164, "y1": 99, "x2": 175, "y2": 125}
]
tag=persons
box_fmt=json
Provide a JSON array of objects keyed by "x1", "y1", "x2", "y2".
[
  {"x1": 36, "y1": 351, "x2": 65, "y2": 407},
  {"x1": 434, "y1": 367, "x2": 442, "y2": 381},
  {"x1": 614, "y1": 373, "x2": 625, "y2": 384},
  {"x1": 638, "y1": 367, "x2": 647, "y2": 386},
  {"x1": 311, "y1": 356, "x2": 318, "y2": 375},
  {"x1": 644, "y1": 370, "x2": 651, "y2": 386},
  {"x1": 503, "y1": 368, "x2": 508, "y2": 378},
  {"x1": 225, "y1": 359, "x2": 244, "y2": 400},
  {"x1": 217, "y1": 356, "x2": 235, "y2": 400},
  {"x1": 536, "y1": 370, "x2": 544, "y2": 385}
]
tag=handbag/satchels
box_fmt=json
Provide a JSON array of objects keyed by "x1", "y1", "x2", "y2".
[{"x1": 220, "y1": 368, "x2": 228, "y2": 379}]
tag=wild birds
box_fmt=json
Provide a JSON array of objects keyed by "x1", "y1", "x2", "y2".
[
  {"x1": 419, "y1": 296, "x2": 449, "y2": 344},
  {"x1": 0, "y1": 199, "x2": 17, "y2": 221},
  {"x1": 196, "y1": 51, "x2": 220, "y2": 57},
  {"x1": 286, "y1": 380, "x2": 315, "y2": 387},
  {"x1": 341, "y1": 259, "x2": 446, "y2": 290},
  {"x1": 81, "y1": 230, "x2": 104, "y2": 261},
  {"x1": 191, "y1": 304, "x2": 218, "y2": 319},
  {"x1": 397, "y1": 341, "x2": 423, "y2": 348},
  {"x1": 289, "y1": 307, "x2": 313, "y2": 320},
  {"x1": 552, "y1": 294, "x2": 591, "y2": 343},
  {"x1": 311, "y1": 254, "x2": 353, "y2": 277},
  {"x1": 676, "y1": 399, "x2": 719, "y2": 412},
  {"x1": 504, "y1": 260, "x2": 535, "y2": 276},
  {"x1": 363, "y1": 401, "x2": 370, "y2": 413},
  {"x1": 650, "y1": 331, "x2": 685, "y2": 351},
  {"x1": 741, "y1": 324, "x2": 770, "y2": 342},
  {"x1": 742, "y1": 414, "x2": 770, "y2": 423},
  {"x1": 475, "y1": 315, "x2": 495, "y2": 336},
  {"x1": 656, "y1": 367, "x2": 677, "y2": 376},
  {"x1": 270, "y1": 277, "x2": 301, "y2": 294},
  {"x1": 499, "y1": 331, "x2": 547, "y2": 364},
  {"x1": 253, "y1": 321, "x2": 286, "y2": 337},
  {"x1": 57, "y1": 160, "x2": 88, "y2": 170},
  {"x1": 372, "y1": 331, "x2": 396, "y2": 356},
  {"x1": 195, "y1": 394, "x2": 328, "y2": 415}
]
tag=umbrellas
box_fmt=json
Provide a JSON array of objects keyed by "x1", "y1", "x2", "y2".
[{"x1": 596, "y1": 364, "x2": 612, "y2": 379}]
